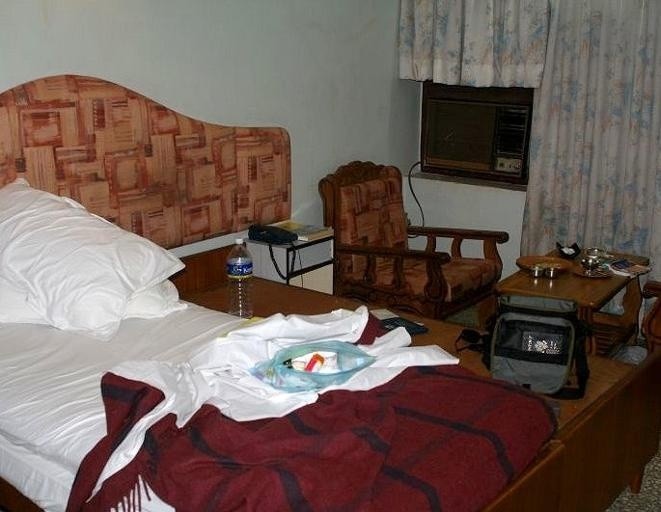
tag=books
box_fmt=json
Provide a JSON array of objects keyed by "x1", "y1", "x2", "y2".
[
  {"x1": 602, "y1": 257, "x2": 653, "y2": 279},
  {"x1": 268, "y1": 220, "x2": 334, "y2": 242}
]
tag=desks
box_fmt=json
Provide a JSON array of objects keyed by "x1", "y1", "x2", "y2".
[{"x1": 495, "y1": 245, "x2": 649, "y2": 355}]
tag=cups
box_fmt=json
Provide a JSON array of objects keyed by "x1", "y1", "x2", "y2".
[{"x1": 528, "y1": 266, "x2": 559, "y2": 278}]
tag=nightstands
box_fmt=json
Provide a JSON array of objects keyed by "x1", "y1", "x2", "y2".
[{"x1": 243, "y1": 219, "x2": 338, "y2": 295}]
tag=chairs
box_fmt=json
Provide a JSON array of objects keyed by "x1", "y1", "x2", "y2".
[{"x1": 315, "y1": 158, "x2": 509, "y2": 321}]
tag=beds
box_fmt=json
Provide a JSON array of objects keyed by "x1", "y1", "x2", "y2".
[{"x1": 0, "y1": 243, "x2": 658, "y2": 512}]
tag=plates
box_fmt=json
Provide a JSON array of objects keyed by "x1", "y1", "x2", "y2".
[{"x1": 515, "y1": 256, "x2": 572, "y2": 272}]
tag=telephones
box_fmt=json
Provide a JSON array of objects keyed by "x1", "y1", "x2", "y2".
[{"x1": 248, "y1": 224, "x2": 298, "y2": 244}]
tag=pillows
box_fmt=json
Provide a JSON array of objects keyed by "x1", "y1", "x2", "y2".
[{"x1": 0, "y1": 176, "x2": 189, "y2": 341}]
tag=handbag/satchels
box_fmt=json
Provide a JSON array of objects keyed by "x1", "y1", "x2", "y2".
[{"x1": 483, "y1": 294, "x2": 593, "y2": 401}]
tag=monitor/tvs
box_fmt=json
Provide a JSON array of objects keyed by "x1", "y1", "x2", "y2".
[{"x1": 419, "y1": 97, "x2": 530, "y2": 179}]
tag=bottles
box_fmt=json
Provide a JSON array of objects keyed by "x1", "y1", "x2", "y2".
[
  {"x1": 226, "y1": 238, "x2": 253, "y2": 319},
  {"x1": 305, "y1": 353, "x2": 324, "y2": 373}
]
